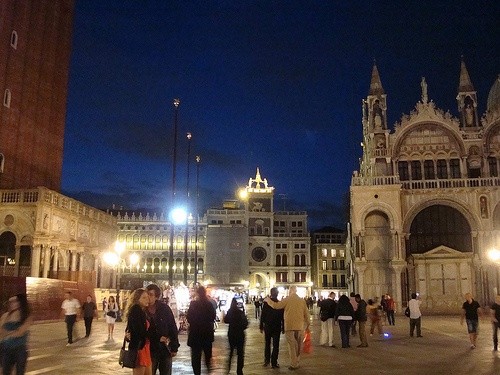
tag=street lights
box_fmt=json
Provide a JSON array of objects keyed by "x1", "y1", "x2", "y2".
[{"x1": 106, "y1": 242, "x2": 139, "y2": 322}]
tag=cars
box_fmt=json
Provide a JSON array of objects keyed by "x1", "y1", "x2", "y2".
[{"x1": 214, "y1": 299, "x2": 246, "y2": 322}]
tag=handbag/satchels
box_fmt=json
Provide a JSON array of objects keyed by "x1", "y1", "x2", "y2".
[
  {"x1": 303, "y1": 328, "x2": 311, "y2": 352},
  {"x1": 119, "y1": 347, "x2": 137, "y2": 369},
  {"x1": 405, "y1": 301, "x2": 410, "y2": 317},
  {"x1": 320, "y1": 312, "x2": 328, "y2": 322}
]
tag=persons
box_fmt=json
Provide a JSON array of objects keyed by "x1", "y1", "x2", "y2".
[
  {"x1": 179, "y1": 289, "x2": 264, "y2": 333},
  {"x1": 259, "y1": 287, "x2": 284, "y2": 369},
  {"x1": 103, "y1": 296, "x2": 118, "y2": 343},
  {"x1": 407, "y1": 293, "x2": 423, "y2": 337},
  {"x1": 102, "y1": 297, "x2": 130, "y2": 319},
  {"x1": 125, "y1": 288, "x2": 153, "y2": 375},
  {"x1": 0, "y1": 292, "x2": 32, "y2": 375},
  {"x1": 60, "y1": 292, "x2": 81, "y2": 347},
  {"x1": 126, "y1": 284, "x2": 180, "y2": 375},
  {"x1": 223, "y1": 298, "x2": 249, "y2": 375},
  {"x1": 186, "y1": 286, "x2": 216, "y2": 375},
  {"x1": 460, "y1": 292, "x2": 483, "y2": 349},
  {"x1": 490, "y1": 294, "x2": 500, "y2": 350},
  {"x1": 263, "y1": 286, "x2": 311, "y2": 370},
  {"x1": 81, "y1": 295, "x2": 97, "y2": 337},
  {"x1": 301, "y1": 291, "x2": 396, "y2": 349}
]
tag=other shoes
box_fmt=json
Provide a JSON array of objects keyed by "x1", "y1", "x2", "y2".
[
  {"x1": 272, "y1": 365, "x2": 280, "y2": 368},
  {"x1": 288, "y1": 366, "x2": 296, "y2": 370},
  {"x1": 264, "y1": 364, "x2": 270, "y2": 366},
  {"x1": 66, "y1": 343, "x2": 71, "y2": 347}
]
